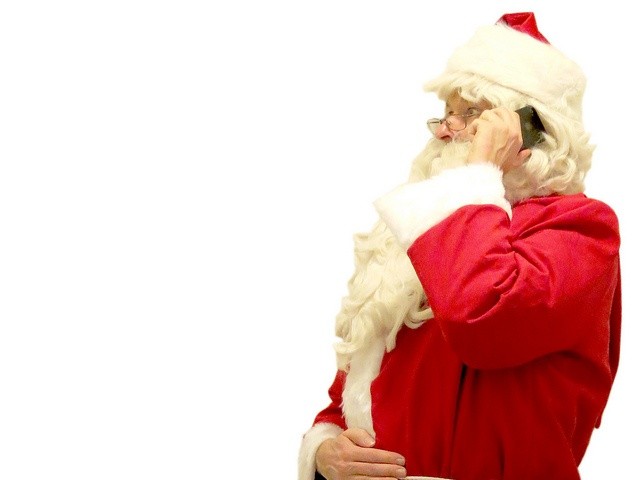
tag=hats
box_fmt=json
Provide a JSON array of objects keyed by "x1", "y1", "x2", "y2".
[{"x1": 448, "y1": 12, "x2": 585, "y2": 120}]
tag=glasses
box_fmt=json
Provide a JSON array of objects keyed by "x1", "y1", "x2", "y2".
[{"x1": 428, "y1": 112, "x2": 483, "y2": 135}]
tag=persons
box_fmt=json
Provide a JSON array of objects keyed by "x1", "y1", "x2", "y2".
[{"x1": 298, "y1": 10, "x2": 622, "y2": 480}]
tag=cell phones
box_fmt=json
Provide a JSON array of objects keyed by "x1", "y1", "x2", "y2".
[{"x1": 515, "y1": 105, "x2": 546, "y2": 154}]
